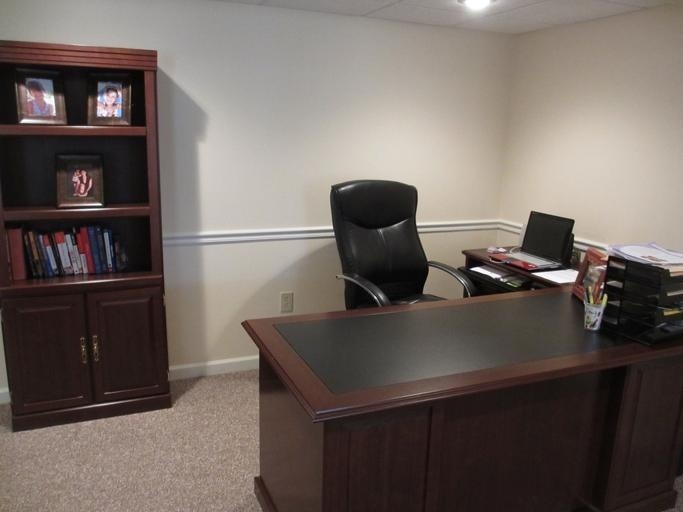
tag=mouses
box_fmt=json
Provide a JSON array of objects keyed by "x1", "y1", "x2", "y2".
[{"x1": 499, "y1": 276, "x2": 514, "y2": 283}]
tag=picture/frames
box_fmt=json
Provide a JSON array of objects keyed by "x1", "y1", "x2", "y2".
[
  {"x1": 11, "y1": 63, "x2": 69, "y2": 129},
  {"x1": 53, "y1": 147, "x2": 109, "y2": 210},
  {"x1": 86, "y1": 70, "x2": 139, "y2": 129}
]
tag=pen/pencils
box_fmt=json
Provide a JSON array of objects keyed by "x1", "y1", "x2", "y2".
[{"x1": 582, "y1": 282, "x2": 608, "y2": 304}]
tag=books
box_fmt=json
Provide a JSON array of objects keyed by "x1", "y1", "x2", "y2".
[
  {"x1": 607, "y1": 240, "x2": 683, "y2": 316},
  {"x1": 6, "y1": 224, "x2": 129, "y2": 281}
]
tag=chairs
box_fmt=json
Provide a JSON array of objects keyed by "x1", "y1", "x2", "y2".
[{"x1": 327, "y1": 174, "x2": 476, "y2": 312}]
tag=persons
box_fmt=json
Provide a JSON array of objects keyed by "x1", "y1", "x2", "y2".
[
  {"x1": 97, "y1": 86, "x2": 122, "y2": 117},
  {"x1": 27, "y1": 80, "x2": 54, "y2": 115},
  {"x1": 72, "y1": 168, "x2": 93, "y2": 197}
]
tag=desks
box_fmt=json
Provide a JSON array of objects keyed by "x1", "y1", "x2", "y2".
[{"x1": 239, "y1": 243, "x2": 683, "y2": 510}]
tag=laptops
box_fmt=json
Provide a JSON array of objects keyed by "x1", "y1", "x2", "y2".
[{"x1": 489, "y1": 210, "x2": 575, "y2": 272}]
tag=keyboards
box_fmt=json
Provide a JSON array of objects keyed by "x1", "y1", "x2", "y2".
[{"x1": 470, "y1": 265, "x2": 515, "y2": 279}]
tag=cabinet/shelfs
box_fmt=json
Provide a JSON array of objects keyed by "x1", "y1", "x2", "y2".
[{"x1": 0, "y1": 34, "x2": 174, "y2": 434}]
